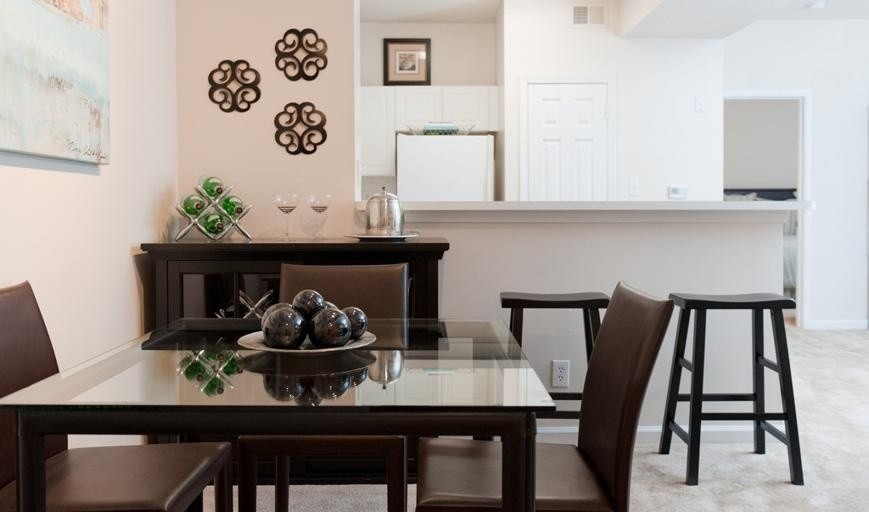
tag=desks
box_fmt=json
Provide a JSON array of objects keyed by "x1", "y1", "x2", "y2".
[
  {"x1": 140, "y1": 318, "x2": 450, "y2": 351},
  {"x1": 139, "y1": 235, "x2": 450, "y2": 330}
]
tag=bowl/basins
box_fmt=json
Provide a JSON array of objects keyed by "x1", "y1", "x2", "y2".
[
  {"x1": 423, "y1": 368, "x2": 456, "y2": 377},
  {"x1": 423, "y1": 120, "x2": 461, "y2": 135}
]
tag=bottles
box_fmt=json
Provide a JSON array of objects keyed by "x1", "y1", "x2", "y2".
[
  {"x1": 219, "y1": 195, "x2": 243, "y2": 217},
  {"x1": 184, "y1": 346, "x2": 245, "y2": 398},
  {"x1": 184, "y1": 195, "x2": 205, "y2": 215},
  {"x1": 199, "y1": 214, "x2": 224, "y2": 236},
  {"x1": 200, "y1": 176, "x2": 224, "y2": 197}
]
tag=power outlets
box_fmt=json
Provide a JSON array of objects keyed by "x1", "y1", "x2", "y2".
[{"x1": 551, "y1": 360, "x2": 570, "y2": 387}]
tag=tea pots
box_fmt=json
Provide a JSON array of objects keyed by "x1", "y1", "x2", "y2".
[
  {"x1": 354, "y1": 185, "x2": 405, "y2": 236},
  {"x1": 367, "y1": 346, "x2": 406, "y2": 390}
]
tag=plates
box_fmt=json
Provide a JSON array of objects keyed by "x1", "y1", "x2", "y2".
[
  {"x1": 345, "y1": 232, "x2": 419, "y2": 240},
  {"x1": 236, "y1": 329, "x2": 377, "y2": 355},
  {"x1": 235, "y1": 351, "x2": 378, "y2": 378}
]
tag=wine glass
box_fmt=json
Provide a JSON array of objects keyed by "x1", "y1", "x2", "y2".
[
  {"x1": 273, "y1": 192, "x2": 300, "y2": 242},
  {"x1": 305, "y1": 191, "x2": 333, "y2": 243}
]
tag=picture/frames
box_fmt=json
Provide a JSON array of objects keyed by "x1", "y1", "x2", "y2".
[{"x1": 382, "y1": 38, "x2": 431, "y2": 86}]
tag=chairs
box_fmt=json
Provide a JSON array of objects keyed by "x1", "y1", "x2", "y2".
[
  {"x1": 0, "y1": 280, "x2": 233, "y2": 510},
  {"x1": 415, "y1": 281, "x2": 675, "y2": 512},
  {"x1": 236, "y1": 263, "x2": 409, "y2": 512}
]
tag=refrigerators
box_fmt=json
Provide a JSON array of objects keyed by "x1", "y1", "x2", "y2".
[{"x1": 395, "y1": 132, "x2": 498, "y2": 202}]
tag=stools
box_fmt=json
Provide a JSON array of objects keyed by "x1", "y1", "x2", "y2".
[
  {"x1": 499, "y1": 290, "x2": 609, "y2": 364},
  {"x1": 659, "y1": 292, "x2": 805, "y2": 486}
]
